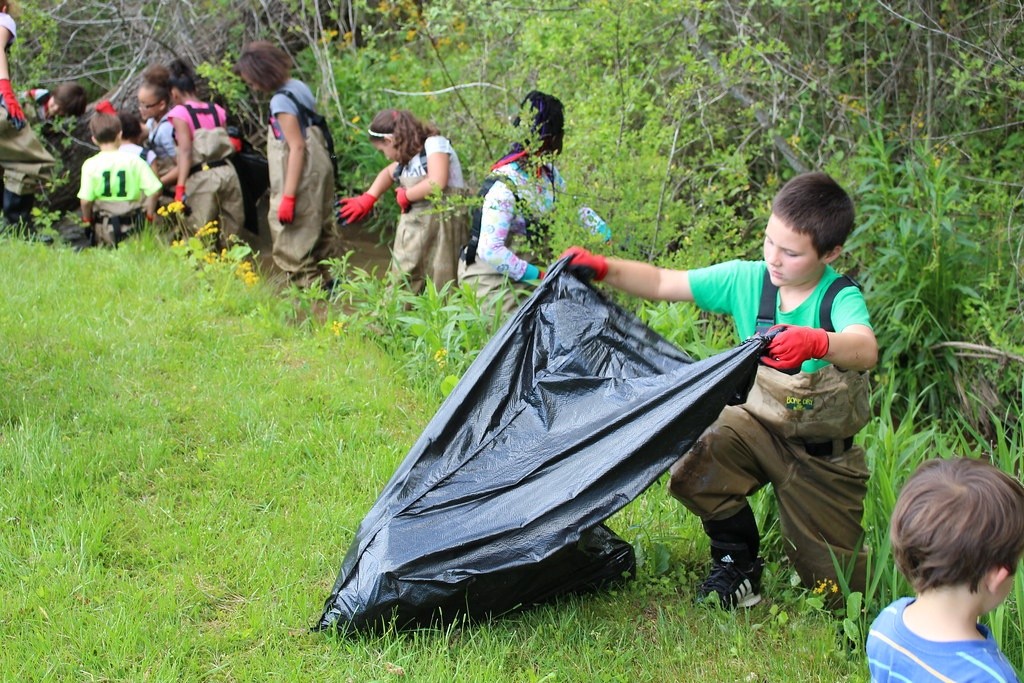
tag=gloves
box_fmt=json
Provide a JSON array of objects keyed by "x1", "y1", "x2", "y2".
[
  {"x1": 394, "y1": 186, "x2": 411, "y2": 213},
  {"x1": 759, "y1": 324, "x2": 829, "y2": 370},
  {"x1": 335, "y1": 192, "x2": 378, "y2": 229},
  {"x1": 0, "y1": 79, "x2": 26, "y2": 131},
  {"x1": 278, "y1": 195, "x2": 297, "y2": 224},
  {"x1": 560, "y1": 246, "x2": 608, "y2": 282},
  {"x1": 96, "y1": 101, "x2": 116, "y2": 116}
]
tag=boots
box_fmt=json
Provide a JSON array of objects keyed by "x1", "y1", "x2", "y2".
[{"x1": 3, "y1": 188, "x2": 53, "y2": 243}]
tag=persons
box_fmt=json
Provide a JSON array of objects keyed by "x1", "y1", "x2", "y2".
[
  {"x1": 197, "y1": 85, "x2": 270, "y2": 237},
  {"x1": 866, "y1": 458, "x2": 1024, "y2": 683},
  {"x1": 166, "y1": 59, "x2": 244, "y2": 251},
  {"x1": 0, "y1": 0, "x2": 56, "y2": 246},
  {"x1": 457, "y1": 89, "x2": 612, "y2": 330},
  {"x1": 19, "y1": 83, "x2": 85, "y2": 120},
  {"x1": 77, "y1": 67, "x2": 178, "y2": 249},
  {"x1": 334, "y1": 108, "x2": 471, "y2": 305},
  {"x1": 561, "y1": 172, "x2": 878, "y2": 620},
  {"x1": 234, "y1": 40, "x2": 340, "y2": 299}
]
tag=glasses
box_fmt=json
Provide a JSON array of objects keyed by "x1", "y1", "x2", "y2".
[{"x1": 137, "y1": 102, "x2": 160, "y2": 108}]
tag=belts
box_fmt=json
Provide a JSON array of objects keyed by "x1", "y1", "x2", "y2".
[
  {"x1": 187, "y1": 159, "x2": 226, "y2": 176},
  {"x1": 804, "y1": 436, "x2": 854, "y2": 456}
]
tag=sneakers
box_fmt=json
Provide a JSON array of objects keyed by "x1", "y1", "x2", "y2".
[{"x1": 692, "y1": 554, "x2": 765, "y2": 609}]
